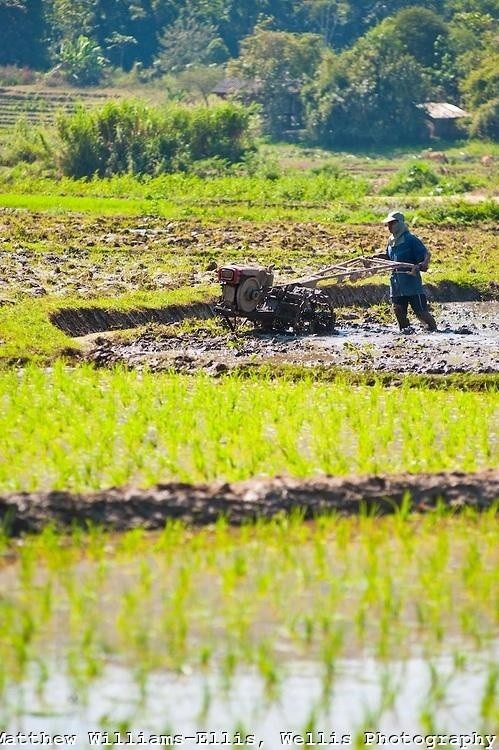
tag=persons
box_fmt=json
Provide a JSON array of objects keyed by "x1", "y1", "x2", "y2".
[{"x1": 365, "y1": 211, "x2": 437, "y2": 332}]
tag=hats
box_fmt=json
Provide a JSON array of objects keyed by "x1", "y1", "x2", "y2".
[{"x1": 380, "y1": 212, "x2": 405, "y2": 224}]
